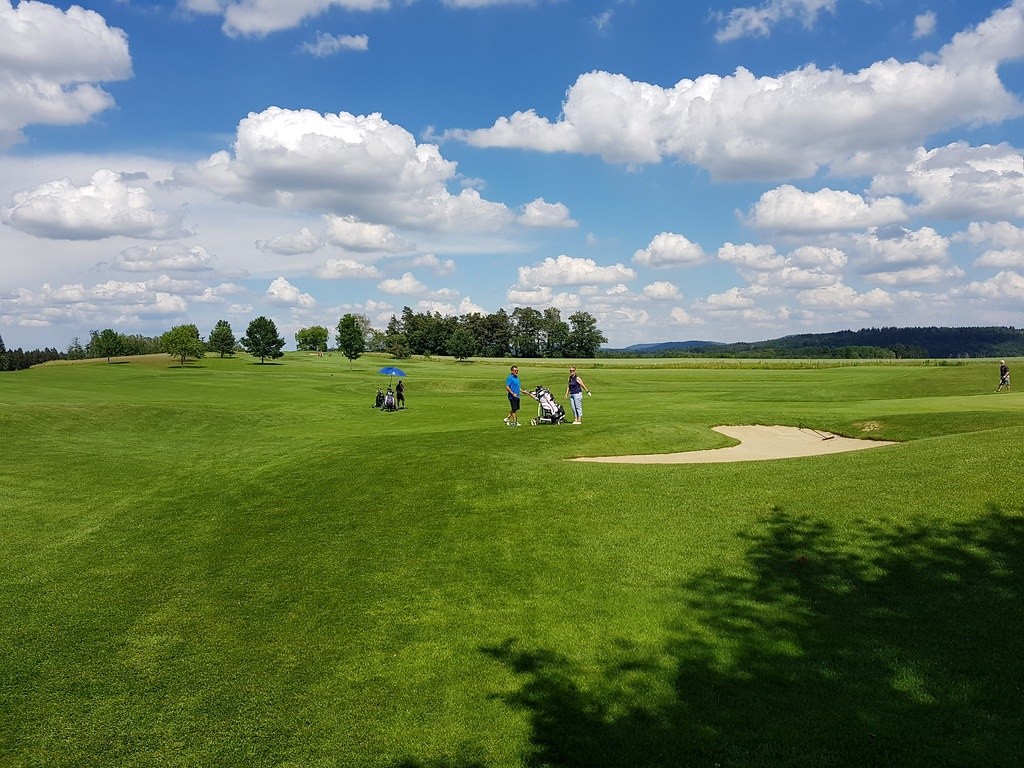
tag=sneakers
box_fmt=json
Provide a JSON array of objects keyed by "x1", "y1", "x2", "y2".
[
  {"x1": 511, "y1": 422, "x2": 521, "y2": 427},
  {"x1": 504, "y1": 418, "x2": 510, "y2": 425}
]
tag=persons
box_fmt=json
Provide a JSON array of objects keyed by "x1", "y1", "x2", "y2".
[
  {"x1": 395, "y1": 379, "x2": 405, "y2": 407},
  {"x1": 503, "y1": 366, "x2": 526, "y2": 426},
  {"x1": 995, "y1": 359, "x2": 1010, "y2": 392},
  {"x1": 562, "y1": 367, "x2": 592, "y2": 425}
]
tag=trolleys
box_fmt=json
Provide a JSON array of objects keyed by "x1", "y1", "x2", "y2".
[
  {"x1": 380, "y1": 386, "x2": 399, "y2": 412},
  {"x1": 524, "y1": 384, "x2": 567, "y2": 427},
  {"x1": 372, "y1": 389, "x2": 385, "y2": 408}
]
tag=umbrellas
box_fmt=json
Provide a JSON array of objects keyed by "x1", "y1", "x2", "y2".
[{"x1": 379, "y1": 366, "x2": 406, "y2": 387}]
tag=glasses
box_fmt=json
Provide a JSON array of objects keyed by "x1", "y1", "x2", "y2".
[{"x1": 570, "y1": 370, "x2": 575, "y2": 372}]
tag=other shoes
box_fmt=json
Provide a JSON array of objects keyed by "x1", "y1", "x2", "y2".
[{"x1": 572, "y1": 421, "x2": 581, "y2": 424}]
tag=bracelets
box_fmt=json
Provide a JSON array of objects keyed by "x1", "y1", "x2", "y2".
[{"x1": 586, "y1": 391, "x2": 590, "y2": 394}]
